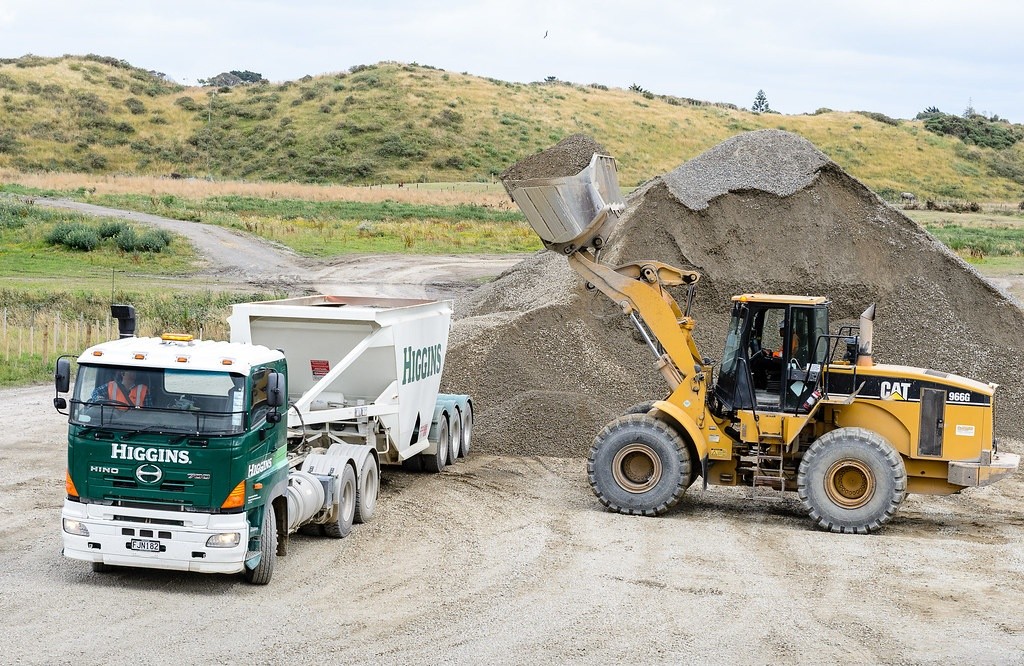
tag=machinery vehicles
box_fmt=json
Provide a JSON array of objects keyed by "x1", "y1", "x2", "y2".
[{"x1": 499, "y1": 153, "x2": 1021, "y2": 535}]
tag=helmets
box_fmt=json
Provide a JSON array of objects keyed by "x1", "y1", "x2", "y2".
[{"x1": 779, "y1": 320, "x2": 796, "y2": 329}]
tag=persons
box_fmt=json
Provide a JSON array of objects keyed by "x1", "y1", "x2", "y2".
[
  {"x1": 87, "y1": 370, "x2": 153, "y2": 410},
  {"x1": 761, "y1": 318, "x2": 799, "y2": 361}
]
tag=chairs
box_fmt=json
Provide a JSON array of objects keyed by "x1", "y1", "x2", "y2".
[
  {"x1": 191, "y1": 395, "x2": 228, "y2": 416},
  {"x1": 765, "y1": 332, "x2": 808, "y2": 380}
]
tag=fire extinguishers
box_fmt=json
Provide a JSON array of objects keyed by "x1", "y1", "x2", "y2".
[{"x1": 803, "y1": 388, "x2": 822, "y2": 410}]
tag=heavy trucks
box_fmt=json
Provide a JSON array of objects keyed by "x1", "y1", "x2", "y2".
[{"x1": 43, "y1": 292, "x2": 476, "y2": 586}]
